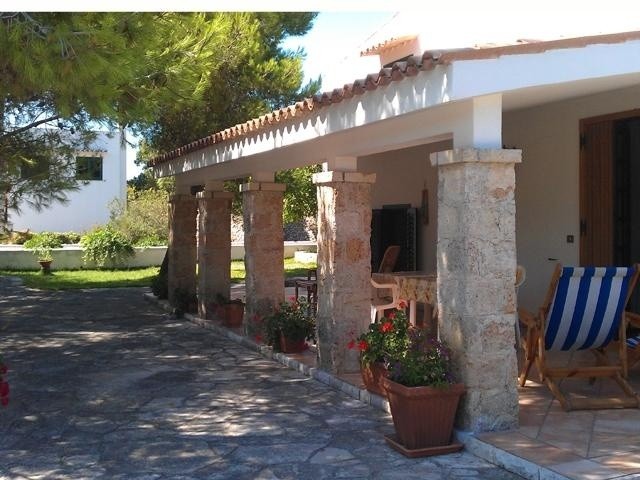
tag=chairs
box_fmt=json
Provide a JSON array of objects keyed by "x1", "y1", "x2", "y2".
[
  {"x1": 295, "y1": 269, "x2": 318, "y2": 303},
  {"x1": 516, "y1": 264, "x2": 526, "y2": 348},
  {"x1": 589, "y1": 310, "x2": 640, "y2": 388},
  {"x1": 517, "y1": 262, "x2": 640, "y2": 413},
  {"x1": 370, "y1": 277, "x2": 399, "y2": 324}
]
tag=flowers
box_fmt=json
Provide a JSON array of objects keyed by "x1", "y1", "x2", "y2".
[
  {"x1": 383, "y1": 326, "x2": 457, "y2": 387},
  {"x1": 254, "y1": 295, "x2": 317, "y2": 354},
  {"x1": 347, "y1": 299, "x2": 409, "y2": 366}
]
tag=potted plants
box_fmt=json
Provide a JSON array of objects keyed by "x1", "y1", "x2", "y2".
[
  {"x1": 224, "y1": 298, "x2": 245, "y2": 328},
  {"x1": 24, "y1": 231, "x2": 64, "y2": 274}
]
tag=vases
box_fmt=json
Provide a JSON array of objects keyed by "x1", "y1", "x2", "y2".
[
  {"x1": 359, "y1": 364, "x2": 387, "y2": 396},
  {"x1": 278, "y1": 327, "x2": 306, "y2": 353},
  {"x1": 382, "y1": 376, "x2": 466, "y2": 450}
]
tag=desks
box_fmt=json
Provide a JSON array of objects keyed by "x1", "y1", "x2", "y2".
[{"x1": 371, "y1": 270, "x2": 438, "y2": 328}]
